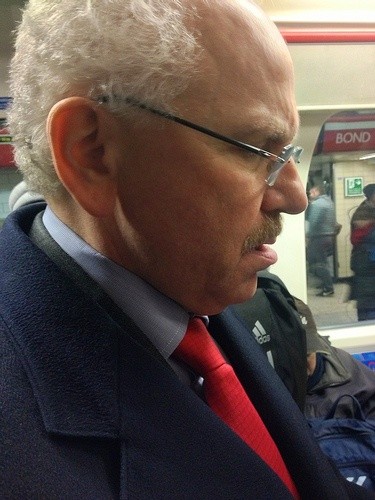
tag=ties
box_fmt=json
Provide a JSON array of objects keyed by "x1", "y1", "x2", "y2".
[{"x1": 170, "y1": 317, "x2": 304, "y2": 500}]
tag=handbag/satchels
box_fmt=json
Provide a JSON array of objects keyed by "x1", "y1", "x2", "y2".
[{"x1": 303, "y1": 391, "x2": 375, "y2": 497}]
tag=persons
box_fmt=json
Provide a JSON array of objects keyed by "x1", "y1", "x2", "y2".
[
  {"x1": 305, "y1": 183, "x2": 375, "y2": 322},
  {"x1": 0, "y1": 1, "x2": 364, "y2": 500},
  {"x1": 292, "y1": 295, "x2": 375, "y2": 424}
]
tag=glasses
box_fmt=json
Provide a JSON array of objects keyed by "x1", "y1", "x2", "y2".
[{"x1": 86, "y1": 94, "x2": 303, "y2": 188}]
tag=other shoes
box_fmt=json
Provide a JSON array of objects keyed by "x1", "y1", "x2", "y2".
[{"x1": 316, "y1": 287, "x2": 334, "y2": 297}]
